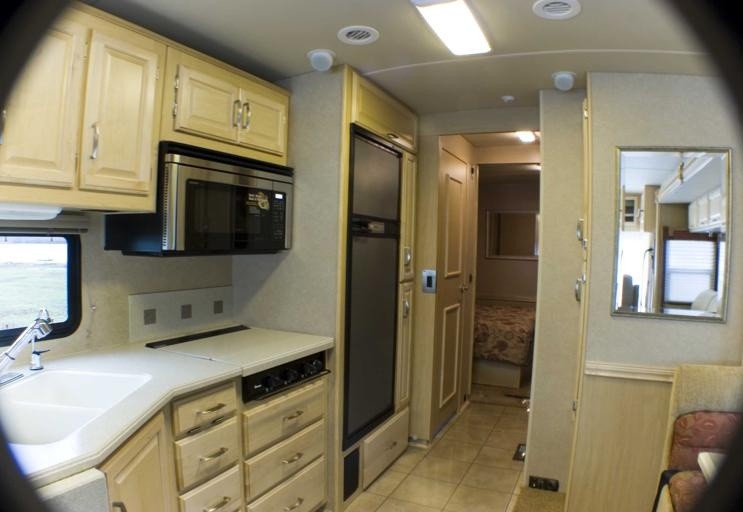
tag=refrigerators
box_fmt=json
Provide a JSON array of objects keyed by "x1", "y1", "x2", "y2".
[{"x1": 342, "y1": 117, "x2": 404, "y2": 450}]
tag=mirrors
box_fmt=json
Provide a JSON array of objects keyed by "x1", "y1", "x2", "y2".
[{"x1": 609, "y1": 145, "x2": 732, "y2": 326}]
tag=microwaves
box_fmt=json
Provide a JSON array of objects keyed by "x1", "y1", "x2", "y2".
[{"x1": 100, "y1": 141, "x2": 297, "y2": 260}]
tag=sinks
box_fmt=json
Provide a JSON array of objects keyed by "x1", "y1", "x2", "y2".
[{"x1": 1, "y1": 371, "x2": 153, "y2": 444}]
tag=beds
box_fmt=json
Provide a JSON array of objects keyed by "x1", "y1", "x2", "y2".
[{"x1": 472, "y1": 304, "x2": 535, "y2": 389}]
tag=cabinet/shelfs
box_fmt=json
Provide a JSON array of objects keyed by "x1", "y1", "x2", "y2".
[
  {"x1": 350, "y1": 68, "x2": 419, "y2": 156},
  {"x1": 620, "y1": 184, "x2": 660, "y2": 233},
  {"x1": 687, "y1": 184, "x2": 725, "y2": 234},
  {"x1": 0, "y1": 0, "x2": 168, "y2": 216},
  {"x1": 161, "y1": 37, "x2": 292, "y2": 168},
  {"x1": 101, "y1": 376, "x2": 329, "y2": 512}
]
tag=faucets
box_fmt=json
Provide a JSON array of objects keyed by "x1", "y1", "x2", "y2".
[
  {"x1": 0, "y1": 317, "x2": 53, "y2": 375},
  {"x1": 30, "y1": 309, "x2": 51, "y2": 371}
]
